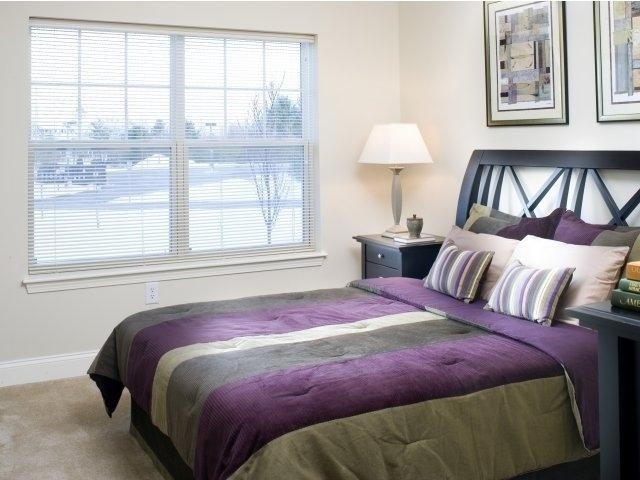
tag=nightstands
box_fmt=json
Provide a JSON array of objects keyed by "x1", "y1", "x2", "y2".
[{"x1": 353, "y1": 234, "x2": 446, "y2": 280}]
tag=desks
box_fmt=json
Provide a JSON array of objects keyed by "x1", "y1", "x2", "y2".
[{"x1": 565, "y1": 303, "x2": 639, "y2": 480}]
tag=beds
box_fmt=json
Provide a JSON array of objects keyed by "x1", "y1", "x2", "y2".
[{"x1": 85, "y1": 151, "x2": 640, "y2": 479}]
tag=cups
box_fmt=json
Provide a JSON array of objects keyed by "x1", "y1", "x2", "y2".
[{"x1": 406, "y1": 218, "x2": 424, "y2": 239}]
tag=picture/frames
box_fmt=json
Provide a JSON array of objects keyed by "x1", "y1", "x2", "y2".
[
  {"x1": 484, "y1": 0, "x2": 569, "y2": 126},
  {"x1": 593, "y1": 0, "x2": 640, "y2": 123}
]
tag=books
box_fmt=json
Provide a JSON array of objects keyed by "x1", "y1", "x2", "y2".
[
  {"x1": 394, "y1": 233, "x2": 436, "y2": 244},
  {"x1": 619, "y1": 278, "x2": 640, "y2": 294},
  {"x1": 610, "y1": 288, "x2": 640, "y2": 312},
  {"x1": 626, "y1": 261, "x2": 640, "y2": 281}
]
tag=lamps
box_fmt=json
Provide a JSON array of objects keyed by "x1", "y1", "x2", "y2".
[{"x1": 356, "y1": 123, "x2": 438, "y2": 240}]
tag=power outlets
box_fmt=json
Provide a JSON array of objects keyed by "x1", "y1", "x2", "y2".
[{"x1": 144, "y1": 280, "x2": 159, "y2": 307}]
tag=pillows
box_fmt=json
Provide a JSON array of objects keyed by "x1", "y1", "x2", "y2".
[
  {"x1": 423, "y1": 237, "x2": 494, "y2": 302},
  {"x1": 552, "y1": 206, "x2": 639, "y2": 260},
  {"x1": 486, "y1": 233, "x2": 630, "y2": 327},
  {"x1": 485, "y1": 261, "x2": 577, "y2": 329},
  {"x1": 449, "y1": 223, "x2": 518, "y2": 303},
  {"x1": 464, "y1": 201, "x2": 561, "y2": 241}
]
